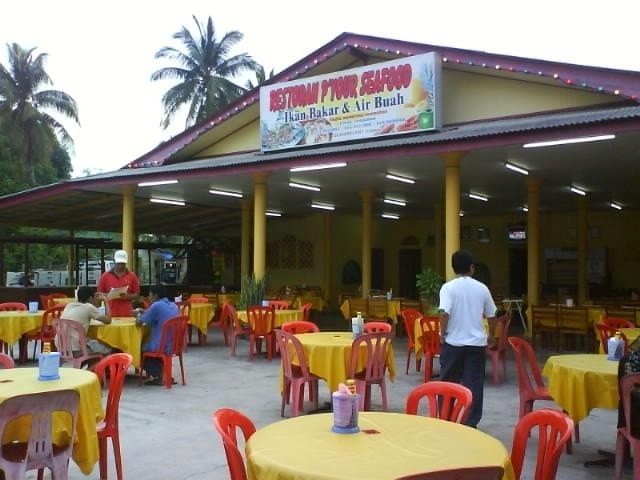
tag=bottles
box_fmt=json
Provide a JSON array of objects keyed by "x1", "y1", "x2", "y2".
[
  {"x1": 42, "y1": 342, "x2": 50, "y2": 354},
  {"x1": 357, "y1": 312, "x2": 361, "y2": 319},
  {"x1": 615, "y1": 332, "x2": 622, "y2": 341},
  {"x1": 346, "y1": 379, "x2": 356, "y2": 426}
]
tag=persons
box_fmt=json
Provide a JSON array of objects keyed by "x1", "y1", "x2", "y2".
[
  {"x1": 438, "y1": 250, "x2": 498, "y2": 429},
  {"x1": 138, "y1": 284, "x2": 179, "y2": 385},
  {"x1": 306, "y1": 287, "x2": 349, "y2": 333},
  {"x1": 617, "y1": 348, "x2": 640, "y2": 464},
  {"x1": 53, "y1": 286, "x2": 112, "y2": 380},
  {"x1": 97, "y1": 250, "x2": 140, "y2": 317}
]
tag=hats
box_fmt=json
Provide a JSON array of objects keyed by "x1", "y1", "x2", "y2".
[{"x1": 115, "y1": 251, "x2": 128, "y2": 264}]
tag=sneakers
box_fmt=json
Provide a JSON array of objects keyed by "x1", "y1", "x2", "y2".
[{"x1": 144, "y1": 377, "x2": 174, "y2": 385}]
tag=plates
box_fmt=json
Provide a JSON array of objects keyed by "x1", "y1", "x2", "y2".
[{"x1": 551, "y1": 303, "x2": 575, "y2": 307}]
